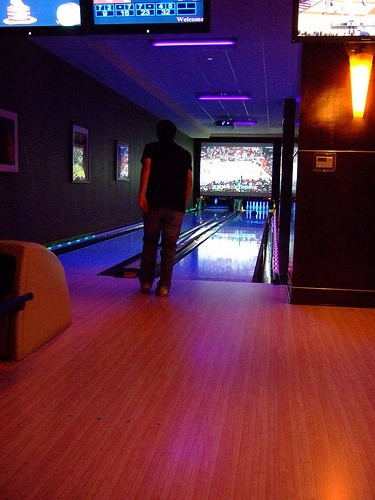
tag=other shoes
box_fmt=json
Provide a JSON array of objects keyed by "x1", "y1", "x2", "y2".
[
  {"x1": 156, "y1": 286, "x2": 168, "y2": 296},
  {"x1": 141, "y1": 282, "x2": 152, "y2": 290}
]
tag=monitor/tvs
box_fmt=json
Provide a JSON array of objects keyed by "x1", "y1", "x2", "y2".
[{"x1": 0, "y1": 0, "x2": 210, "y2": 36}]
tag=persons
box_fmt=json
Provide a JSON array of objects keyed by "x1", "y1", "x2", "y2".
[{"x1": 140, "y1": 120, "x2": 192, "y2": 295}]
